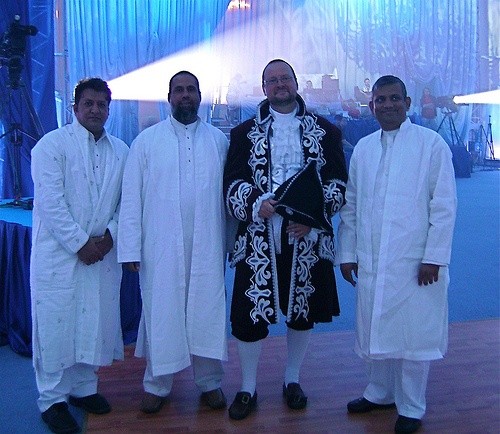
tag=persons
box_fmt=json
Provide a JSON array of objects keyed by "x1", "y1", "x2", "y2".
[
  {"x1": 28, "y1": 76, "x2": 132, "y2": 434},
  {"x1": 227, "y1": 72, "x2": 246, "y2": 126},
  {"x1": 421, "y1": 87, "x2": 439, "y2": 132},
  {"x1": 115, "y1": 68, "x2": 236, "y2": 411},
  {"x1": 301, "y1": 81, "x2": 314, "y2": 102},
  {"x1": 223, "y1": 58, "x2": 349, "y2": 419},
  {"x1": 339, "y1": 75, "x2": 456, "y2": 433},
  {"x1": 361, "y1": 77, "x2": 373, "y2": 93}
]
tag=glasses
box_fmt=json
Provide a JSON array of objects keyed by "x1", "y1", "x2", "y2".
[{"x1": 263, "y1": 75, "x2": 294, "y2": 85}]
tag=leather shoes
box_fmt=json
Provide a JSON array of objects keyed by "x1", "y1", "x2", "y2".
[
  {"x1": 282, "y1": 380, "x2": 308, "y2": 410},
  {"x1": 347, "y1": 397, "x2": 396, "y2": 412},
  {"x1": 395, "y1": 415, "x2": 422, "y2": 434},
  {"x1": 141, "y1": 392, "x2": 164, "y2": 413},
  {"x1": 204, "y1": 388, "x2": 227, "y2": 410},
  {"x1": 228, "y1": 388, "x2": 257, "y2": 419}
]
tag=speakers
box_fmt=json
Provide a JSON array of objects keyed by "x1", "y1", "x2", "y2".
[{"x1": 449, "y1": 145, "x2": 471, "y2": 178}]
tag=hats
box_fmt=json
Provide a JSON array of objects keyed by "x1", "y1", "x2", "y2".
[{"x1": 270, "y1": 150, "x2": 336, "y2": 238}]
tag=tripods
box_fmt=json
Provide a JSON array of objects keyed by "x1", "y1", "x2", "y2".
[
  {"x1": 0, "y1": 79, "x2": 46, "y2": 207},
  {"x1": 436, "y1": 112, "x2": 496, "y2": 166}
]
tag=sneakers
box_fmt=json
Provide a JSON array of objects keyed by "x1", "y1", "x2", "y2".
[
  {"x1": 68, "y1": 394, "x2": 112, "y2": 416},
  {"x1": 42, "y1": 403, "x2": 81, "y2": 434}
]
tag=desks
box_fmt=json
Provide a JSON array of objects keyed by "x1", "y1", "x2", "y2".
[{"x1": 0, "y1": 198, "x2": 141, "y2": 356}]
